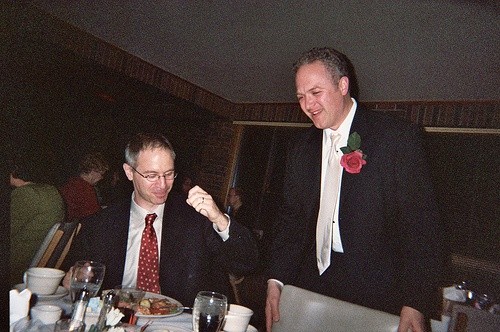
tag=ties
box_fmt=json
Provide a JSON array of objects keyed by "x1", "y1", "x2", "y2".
[
  {"x1": 316, "y1": 134, "x2": 341, "y2": 276},
  {"x1": 137, "y1": 213, "x2": 161, "y2": 295}
]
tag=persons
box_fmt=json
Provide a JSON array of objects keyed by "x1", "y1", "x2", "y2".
[
  {"x1": 58, "y1": 131, "x2": 259, "y2": 320},
  {"x1": 263, "y1": 48, "x2": 444, "y2": 332},
  {"x1": 55, "y1": 145, "x2": 111, "y2": 224},
  {"x1": 6, "y1": 135, "x2": 66, "y2": 247},
  {"x1": 226, "y1": 184, "x2": 257, "y2": 226}
]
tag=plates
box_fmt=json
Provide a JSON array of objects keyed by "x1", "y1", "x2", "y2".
[
  {"x1": 101, "y1": 289, "x2": 185, "y2": 317},
  {"x1": 11, "y1": 283, "x2": 68, "y2": 300}
]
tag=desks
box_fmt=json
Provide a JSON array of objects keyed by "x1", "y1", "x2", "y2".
[{"x1": 10, "y1": 285, "x2": 257, "y2": 332}]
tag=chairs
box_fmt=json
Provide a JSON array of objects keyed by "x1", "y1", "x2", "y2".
[
  {"x1": 267, "y1": 284, "x2": 400, "y2": 332},
  {"x1": 448, "y1": 304, "x2": 500, "y2": 332},
  {"x1": 28, "y1": 220, "x2": 83, "y2": 269}
]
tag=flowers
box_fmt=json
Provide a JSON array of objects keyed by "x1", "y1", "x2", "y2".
[{"x1": 339, "y1": 131, "x2": 368, "y2": 173}]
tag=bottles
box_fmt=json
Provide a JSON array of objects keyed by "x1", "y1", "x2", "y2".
[{"x1": 65, "y1": 286, "x2": 116, "y2": 331}]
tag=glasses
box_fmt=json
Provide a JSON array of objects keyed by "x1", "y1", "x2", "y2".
[{"x1": 128, "y1": 164, "x2": 178, "y2": 182}]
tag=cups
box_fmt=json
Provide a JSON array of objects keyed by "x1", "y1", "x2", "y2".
[
  {"x1": 192, "y1": 291, "x2": 227, "y2": 332},
  {"x1": 23, "y1": 267, "x2": 66, "y2": 295},
  {"x1": 220, "y1": 304, "x2": 255, "y2": 332},
  {"x1": 70, "y1": 260, "x2": 106, "y2": 303},
  {"x1": 55, "y1": 316, "x2": 85, "y2": 332},
  {"x1": 31, "y1": 305, "x2": 62, "y2": 331}
]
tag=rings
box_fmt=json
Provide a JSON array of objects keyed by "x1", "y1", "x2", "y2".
[{"x1": 201, "y1": 197, "x2": 205, "y2": 203}]
